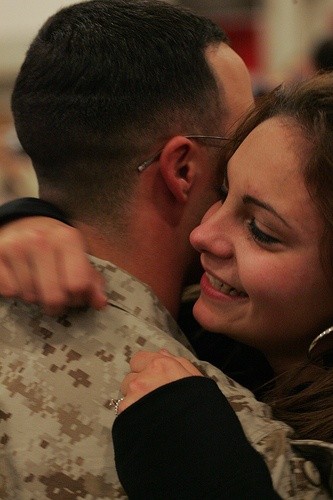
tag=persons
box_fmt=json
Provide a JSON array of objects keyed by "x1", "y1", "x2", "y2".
[
  {"x1": 0, "y1": 0, "x2": 328, "y2": 500},
  {"x1": 0, "y1": 74, "x2": 333, "y2": 500}
]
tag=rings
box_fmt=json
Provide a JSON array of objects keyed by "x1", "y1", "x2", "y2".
[{"x1": 110, "y1": 397, "x2": 123, "y2": 415}]
